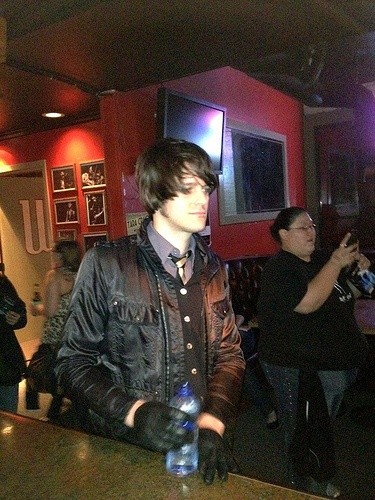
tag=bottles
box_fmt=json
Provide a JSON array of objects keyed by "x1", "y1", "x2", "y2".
[
  {"x1": 31, "y1": 284, "x2": 43, "y2": 316},
  {"x1": 165, "y1": 383, "x2": 200, "y2": 478}
]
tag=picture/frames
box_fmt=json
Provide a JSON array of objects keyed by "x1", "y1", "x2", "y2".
[
  {"x1": 84, "y1": 190, "x2": 107, "y2": 226},
  {"x1": 53, "y1": 195, "x2": 80, "y2": 226},
  {"x1": 81, "y1": 231, "x2": 108, "y2": 254},
  {"x1": 50, "y1": 163, "x2": 78, "y2": 193},
  {"x1": 79, "y1": 158, "x2": 107, "y2": 189},
  {"x1": 57, "y1": 228, "x2": 78, "y2": 240}
]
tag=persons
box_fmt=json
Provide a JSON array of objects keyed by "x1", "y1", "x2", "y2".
[
  {"x1": 25, "y1": 242, "x2": 83, "y2": 410},
  {"x1": 257, "y1": 207, "x2": 369, "y2": 498},
  {"x1": 58, "y1": 166, "x2": 103, "y2": 224},
  {"x1": 53, "y1": 137, "x2": 245, "y2": 486},
  {"x1": 234, "y1": 303, "x2": 279, "y2": 429},
  {"x1": 0, "y1": 273, "x2": 28, "y2": 415}
]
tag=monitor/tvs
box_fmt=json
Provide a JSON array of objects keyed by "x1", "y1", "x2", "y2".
[{"x1": 156, "y1": 87, "x2": 227, "y2": 176}]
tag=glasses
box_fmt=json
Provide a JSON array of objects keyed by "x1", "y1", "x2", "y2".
[{"x1": 288, "y1": 223, "x2": 317, "y2": 232}]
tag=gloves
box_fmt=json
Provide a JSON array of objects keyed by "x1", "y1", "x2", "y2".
[
  {"x1": 133, "y1": 400, "x2": 195, "y2": 452},
  {"x1": 198, "y1": 427, "x2": 237, "y2": 485}
]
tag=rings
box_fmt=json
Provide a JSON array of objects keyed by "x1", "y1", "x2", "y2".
[{"x1": 340, "y1": 244, "x2": 347, "y2": 247}]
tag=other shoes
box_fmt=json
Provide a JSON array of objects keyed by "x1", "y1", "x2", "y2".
[
  {"x1": 267, "y1": 409, "x2": 282, "y2": 430},
  {"x1": 300, "y1": 477, "x2": 341, "y2": 498}
]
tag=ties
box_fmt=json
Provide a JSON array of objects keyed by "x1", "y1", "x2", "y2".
[{"x1": 168, "y1": 251, "x2": 192, "y2": 286}]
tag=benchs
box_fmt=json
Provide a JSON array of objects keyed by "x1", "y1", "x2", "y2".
[{"x1": 226, "y1": 252, "x2": 271, "y2": 321}]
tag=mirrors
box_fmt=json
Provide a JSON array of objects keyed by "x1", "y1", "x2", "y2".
[{"x1": 217, "y1": 119, "x2": 290, "y2": 226}]
